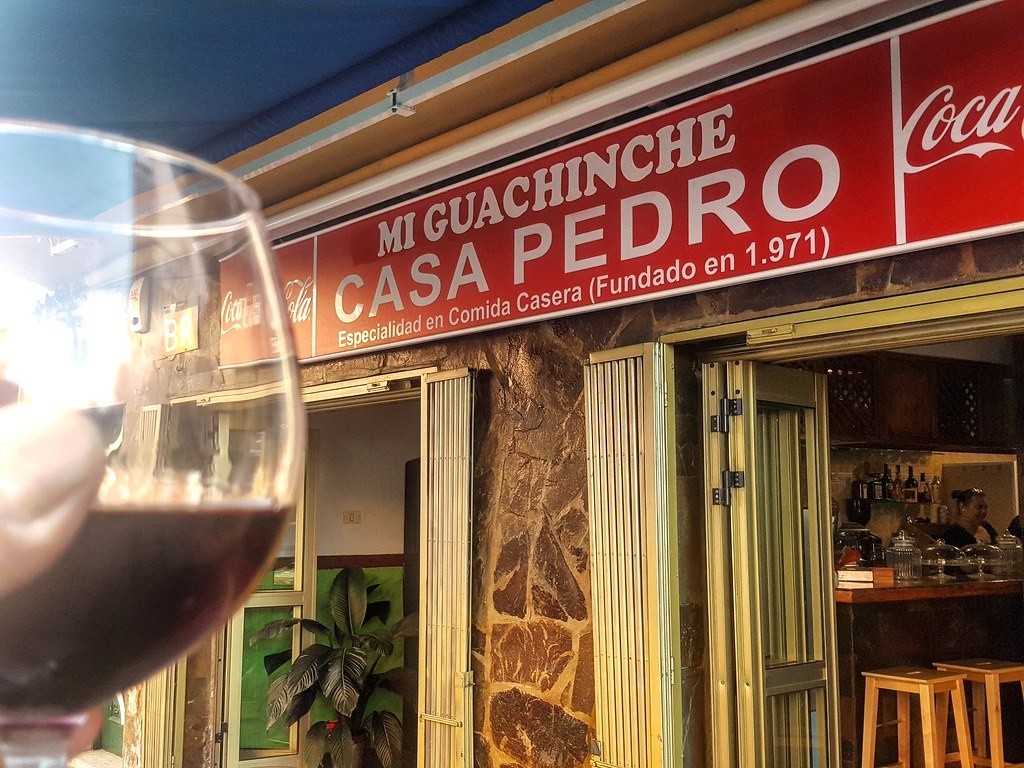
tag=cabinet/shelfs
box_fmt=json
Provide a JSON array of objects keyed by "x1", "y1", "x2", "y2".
[{"x1": 778, "y1": 350, "x2": 1024, "y2": 457}]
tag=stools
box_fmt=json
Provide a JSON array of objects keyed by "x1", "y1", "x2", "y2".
[
  {"x1": 933, "y1": 656, "x2": 1024, "y2": 768},
  {"x1": 860, "y1": 668, "x2": 974, "y2": 768}
]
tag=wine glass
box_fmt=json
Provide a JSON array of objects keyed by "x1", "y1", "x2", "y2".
[{"x1": 0, "y1": 120, "x2": 315, "y2": 768}]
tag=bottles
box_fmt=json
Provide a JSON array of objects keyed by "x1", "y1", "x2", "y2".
[
  {"x1": 882, "y1": 462, "x2": 893, "y2": 499},
  {"x1": 869, "y1": 471, "x2": 883, "y2": 498},
  {"x1": 918, "y1": 472, "x2": 932, "y2": 502},
  {"x1": 892, "y1": 464, "x2": 904, "y2": 500},
  {"x1": 904, "y1": 465, "x2": 918, "y2": 502},
  {"x1": 885, "y1": 529, "x2": 922, "y2": 580}
]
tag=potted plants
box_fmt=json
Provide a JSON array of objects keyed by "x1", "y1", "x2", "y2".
[{"x1": 249, "y1": 565, "x2": 419, "y2": 768}]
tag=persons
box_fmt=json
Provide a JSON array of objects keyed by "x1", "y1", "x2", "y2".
[{"x1": 942, "y1": 489, "x2": 1000, "y2": 548}]
